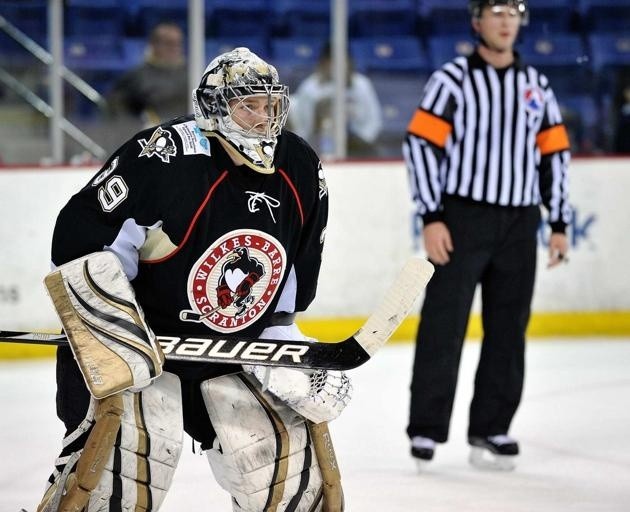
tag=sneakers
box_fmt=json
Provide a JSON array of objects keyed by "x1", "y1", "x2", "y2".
[
  {"x1": 467, "y1": 431, "x2": 519, "y2": 454},
  {"x1": 411, "y1": 436, "x2": 434, "y2": 459}
]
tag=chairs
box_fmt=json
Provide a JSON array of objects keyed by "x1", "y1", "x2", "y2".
[{"x1": 1, "y1": 1, "x2": 629, "y2": 161}]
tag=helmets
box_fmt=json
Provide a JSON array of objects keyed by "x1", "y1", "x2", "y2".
[
  {"x1": 467, "y1": 1, "x2": 530, "y2": 26},
  {"x1": 194, "y1": 47, "x2": 282, "y2": 166}
]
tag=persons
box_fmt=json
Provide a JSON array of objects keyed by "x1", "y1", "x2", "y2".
[
  {"x1": 98, "y1": 21, "x2": 193, "y2": 163},
  {"x1": 288, "y1": 41, "x2": 384, "y2": 160},
  {"x1": 33, "y1": 49, "x2": 339, "y2": 512},
  {"x1": 399, "y1": 0, "x2": 570, "y2": 460}
]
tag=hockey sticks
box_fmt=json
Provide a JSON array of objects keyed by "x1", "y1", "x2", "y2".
[{"x1": 0, "y1": 255, "x2": 436, "y2": 371}]
